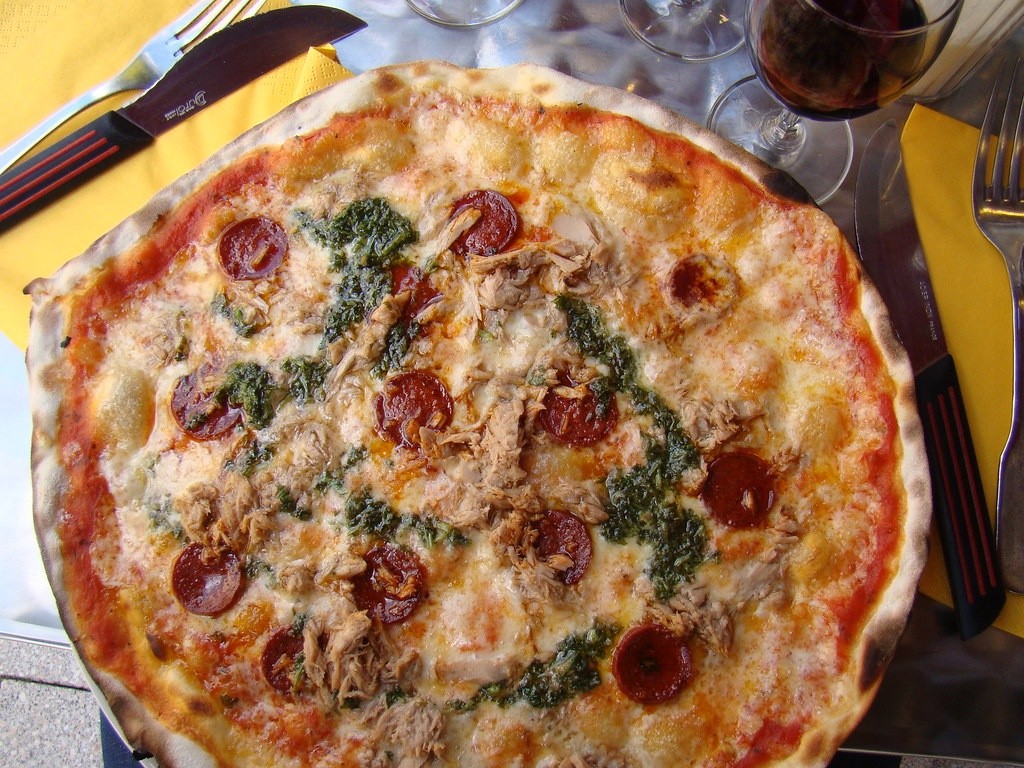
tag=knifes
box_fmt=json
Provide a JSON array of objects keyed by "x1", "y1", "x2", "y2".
[
  {"x1": 854, "y1": 119, "x2": 1006, "y2": 640},
  {"x1": 0, "y1": 5, "x2": 368, "y2": 231}
]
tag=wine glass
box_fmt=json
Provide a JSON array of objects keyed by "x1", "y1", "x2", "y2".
[
  {"x1": 404, "y1": 1, "x2": 524, "y2": 29},
  {"x1": 706, "y1": 0, "x2": 963, "y2": 205},
  {"x1": 619, "y1": 1, "x2": 747, "y2": 62}
]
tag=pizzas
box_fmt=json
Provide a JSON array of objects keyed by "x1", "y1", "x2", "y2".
[{"x1": 22, "y1": 62, "x2": 932, "y2": 768}]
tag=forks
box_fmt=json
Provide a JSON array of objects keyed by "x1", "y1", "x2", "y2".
[
  {"x1": 970, "y1": 56, "x2": 1024, "y2": 597},
  {"x1": 1, "y1": 0, "x2": 269, "y2": 174}
]
